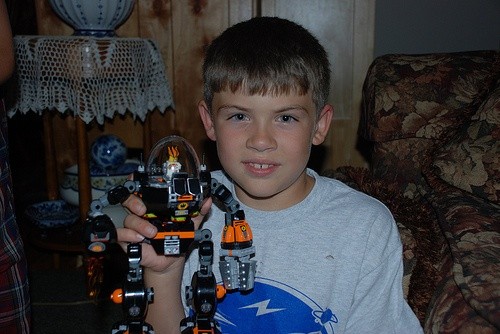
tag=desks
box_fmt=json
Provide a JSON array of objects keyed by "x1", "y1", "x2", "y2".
[{"x1": 9, "y1": 34, "x2": 176, "y2": 295}]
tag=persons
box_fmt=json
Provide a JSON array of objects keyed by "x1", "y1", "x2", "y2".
[
  {"x1": 0, "y1": 0, "x2": 34, "y2": 334},
  {"x1": 115, "y1": 15, "x2": 424, "y2": 333},
  {"x1": 84, "y1": 135, "x2": 258, "y2": 334}
]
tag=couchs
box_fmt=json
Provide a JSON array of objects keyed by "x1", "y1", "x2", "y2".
[{"x1": 319, "y1": 49, "x2": 500, "y2": 333}]
